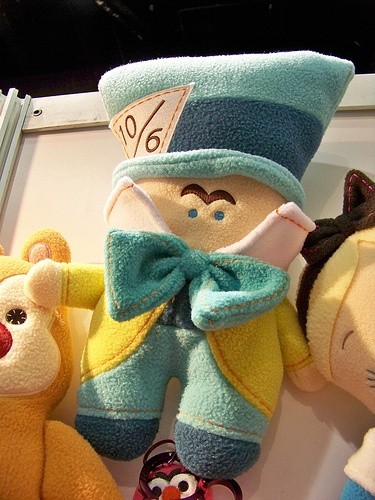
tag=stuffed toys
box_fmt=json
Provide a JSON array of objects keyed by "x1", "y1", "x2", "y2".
[
  {"x1": 0, "y1": 229, "x2": 122, "y2": 500},
  {"x1": 129, "y1": 438, "x2": 243, "y2": 500},
  {"x1": 26, "y1": 49, "x2": 357, "y2": 483},
  {"x1": 300, "y1": 167, "x2": 375, "y2": 500}
]
